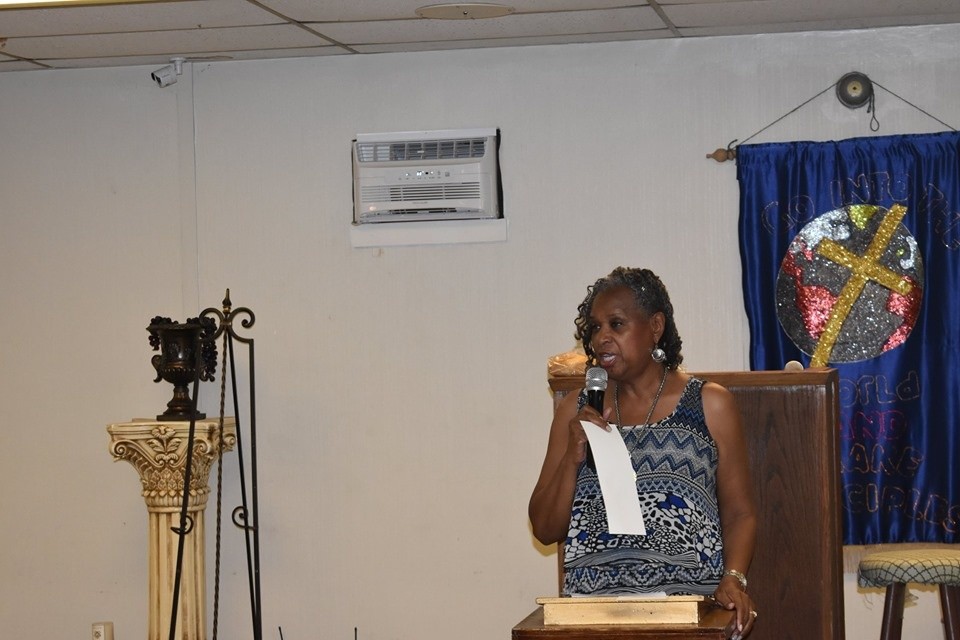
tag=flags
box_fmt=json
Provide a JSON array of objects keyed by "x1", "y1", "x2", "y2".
[{"x1": 735, "y1": 128, "x2": 960, "y2": 545}]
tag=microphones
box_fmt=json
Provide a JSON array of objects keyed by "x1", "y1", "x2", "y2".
[{"x1": 586, "y1": 368, "x2": 608, "y2": 468}]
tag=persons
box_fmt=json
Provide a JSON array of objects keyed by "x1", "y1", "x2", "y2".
[{"x1": 524, "y1": 265, "x2": 756, "y2": 640}]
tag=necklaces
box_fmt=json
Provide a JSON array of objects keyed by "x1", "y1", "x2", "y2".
[{"x1": 615, "y1": 367, "x2": 668, "y2": 458}]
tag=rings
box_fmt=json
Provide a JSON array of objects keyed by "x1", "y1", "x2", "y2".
[{"x1": 749, "y1": 610, "x2": 758, "y2": 619}]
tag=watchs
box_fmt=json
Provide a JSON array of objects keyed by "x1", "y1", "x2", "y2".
[{"x1": 723, "y1": 569, "x2": 748, "y2": 593}]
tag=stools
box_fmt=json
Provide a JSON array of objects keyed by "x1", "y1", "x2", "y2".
[{"x1": 857, "y1": 548, "x2": 960, "y2": 640}]
